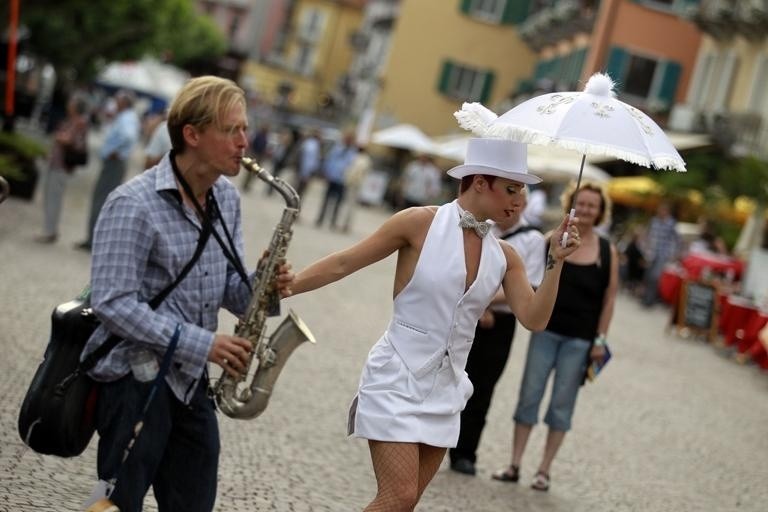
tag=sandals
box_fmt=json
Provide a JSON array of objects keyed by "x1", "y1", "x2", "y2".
[
  {"x1": 531, "y1": 471, "x2": 550, "y2": 491},
  {"x1": 492, "y1": 465, "x2": 519, "y2": 482}
]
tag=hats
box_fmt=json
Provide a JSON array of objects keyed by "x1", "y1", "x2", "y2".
[{"x1": 446, "y1": 138, "x2": 543, "y2": 185}]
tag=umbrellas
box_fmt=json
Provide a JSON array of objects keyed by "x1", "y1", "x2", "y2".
[{"x1": 453, "y1": 72, "x2": 688, "y2": 249}]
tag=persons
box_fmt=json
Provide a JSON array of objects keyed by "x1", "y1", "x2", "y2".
[
  {"x1": 30, "y1": 85, "x2": 724, "y2": 309},
  {"x1": 78, "y1": 74, "x2": 296, "y2": 512},
  {"x1": 493, "y1": 177, "x2": 619, "y2": 492},
  {"x1": 449, "y1": 186, "x2": 546, "y2": 477},
  {"x1": 259, "y1": 137, "x2": 583, "y2": 512}
]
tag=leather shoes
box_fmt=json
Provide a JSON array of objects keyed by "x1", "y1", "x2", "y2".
[{"x1": 450, "y1": 457, "x2": 475, "y2": 475}]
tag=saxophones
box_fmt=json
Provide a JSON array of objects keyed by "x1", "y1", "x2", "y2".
[{"x1": 215, "y1": 158, "x2": 317, "y2": 420}]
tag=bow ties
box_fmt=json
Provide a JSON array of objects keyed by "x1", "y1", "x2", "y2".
[{"x1": 458, "y1": 210, "x2": 491, "y2": 239}]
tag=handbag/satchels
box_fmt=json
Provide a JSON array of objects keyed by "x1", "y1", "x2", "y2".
[{"x1": 63, "y1": 140, "x2": 88, "y2": 172}]
